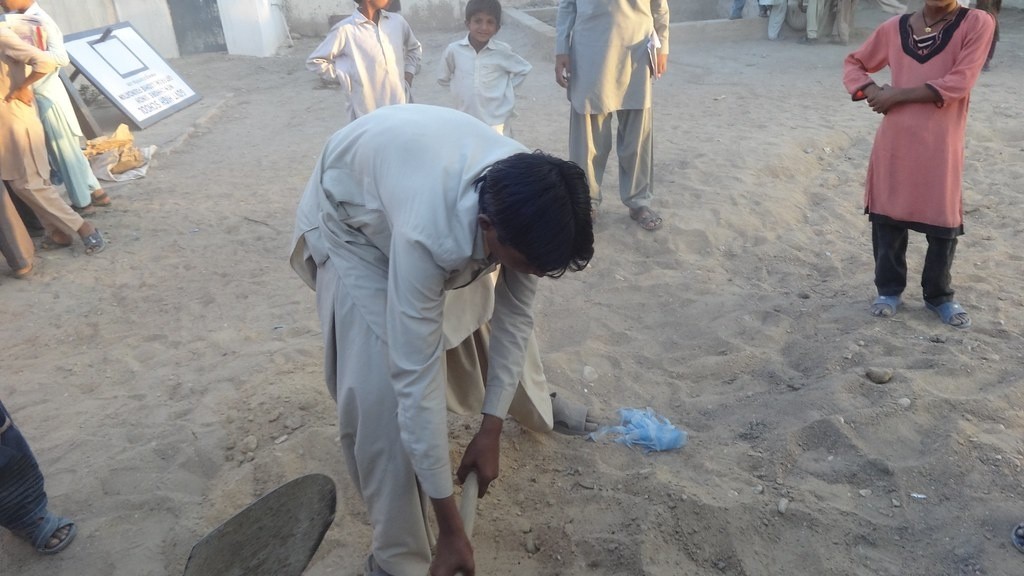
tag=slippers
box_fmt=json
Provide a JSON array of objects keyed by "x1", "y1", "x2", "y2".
[
  {"x1": 631, "y1": 207, "x2": 663, "y2": 230},
  {"x1": 15, "y1": 255, "x2": 44, "y2": 279},
  {"x1": 870, "y1": 294, "x2": 903, "y2": 317},
  {"x1": 82, "y1": 227, "x2": 106, "y2": 255},
  {"x1": 925, "y1": 300, "x2": 971, "y2": 328},
  {"x1": 363, "y1": 554, "x2": 392, "y2": 576},
  {"x1": 38, "y1": 236, "x2": 73, "y2": 251},
  {"x1": 590, "y1": 209, "x2": 597, "y2": 225},
  {"x1": 14, "y1": 510, "x2": 78, "y2": 556},
  {"x1": 547, "y1": 391, "x2": 602, "y2": 436}
]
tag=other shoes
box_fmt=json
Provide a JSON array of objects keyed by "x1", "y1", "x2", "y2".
[
  {"x1": 828, "y1": 36, "x2": 849, "y2": 46},
  {"x1": 797, "y1": 36, "x2": 816, "y2": 46}
]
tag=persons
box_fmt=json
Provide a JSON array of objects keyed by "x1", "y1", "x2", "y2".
[
  {"x1": 437, "y1": 0, "x2": 533, "y2": 140},
  {"x1": 1, "y1": 22, "x2": 107, "y2": 258},
  {"x1": 759, "y1": 0, "x2": 789, "y2": 40},
  {"x1": 840, "y1": 0, "x2": 997, "y2": 330},
  {"x1": 554, "y1": 1, "x2": 671, "y2": 232},
  {"x1": 729, "y1": 0, "x2": 769, "y2": 20},
  {"x1": 305, "y1": 1, "x2": 423, "y2": 122},
  {"x1": 0, "y1": 1, "x2": 110, "y2": 216},
  {"x1": 0, "y1": 399, "x2": 77, "y2": 555},
  {"x1": 797, "y1": 0, "x2": 832, "y2": 45},
  {"x1": 831, "y1": 0, "x2": 859, "y2": 45},
  {"x1": 0, "y1": 174, "x2": 36, "y2": 279},
  {"x1": 289, "y1": 101, "x2": 599, "y2": 576}
]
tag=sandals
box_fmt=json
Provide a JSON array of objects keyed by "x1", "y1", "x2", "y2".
[
  {"x1": 67, "y1": 204, "x2": 96, "y2": 218},
  {"x1": 92, "y1": 192, "x2": 113, "y2": 207}
]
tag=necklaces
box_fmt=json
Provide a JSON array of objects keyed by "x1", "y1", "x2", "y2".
[{"x1": 921, "y1": 8, "x2": 957, "y2": 34}]
